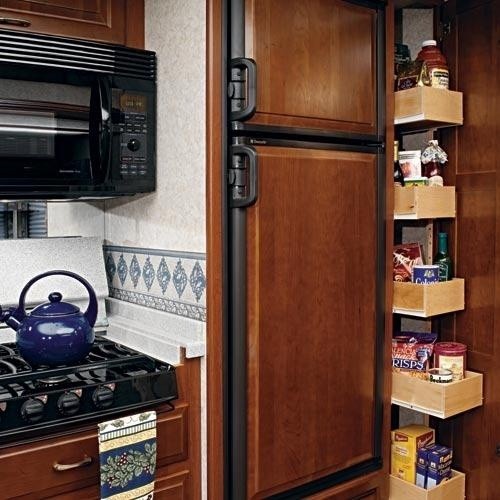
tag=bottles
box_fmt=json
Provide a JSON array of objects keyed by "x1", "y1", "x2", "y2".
[
  {"x1": 433, "y1": 232, "x2": 453, "y2": 282},
  {"x1": 422, "y1": 139, "x2": 446, "y2": 187},
  {"x1": 415, "y1": 39, "x2": 453, "y2": 92},
  {"x1": 393, "y1": 138, "x2": 404, "y2": 188}
]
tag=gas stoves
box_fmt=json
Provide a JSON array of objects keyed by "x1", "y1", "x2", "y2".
[{"x1": 0, "y1": 301, "x2": 180, "y2": 449}]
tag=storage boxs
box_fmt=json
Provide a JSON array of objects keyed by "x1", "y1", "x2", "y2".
[{"x1": 391, "y1": 85, "x2": 483, "y2": 500}]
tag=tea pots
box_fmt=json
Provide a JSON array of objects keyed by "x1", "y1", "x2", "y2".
[{"x1": 0, "y1": 268, "x2": 98, "y2": 368}]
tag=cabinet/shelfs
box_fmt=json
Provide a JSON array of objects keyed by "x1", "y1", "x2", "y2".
[
  {"x1": 206, "y1": 1, "x2": 391, "y2": 500},
  {"x1": 0, "y1": 1, "x2": 144, "y2": 50}
]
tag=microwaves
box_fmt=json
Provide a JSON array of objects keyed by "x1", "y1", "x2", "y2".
[{"x1": 0, "y1": 30, "x2": 158, "y2": 202}]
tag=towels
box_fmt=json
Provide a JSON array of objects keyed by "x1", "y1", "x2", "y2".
[{"x1": 97, "y1": 410, "x2": 157, "y2": 500}]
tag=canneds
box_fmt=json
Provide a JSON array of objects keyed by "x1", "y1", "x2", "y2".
[
  {"x1": 433, "y1": 341, "x2": 468, "y2": 382},
  {"x1": 427, "y1": 368, "x2": 453, "y2": 384}
]
